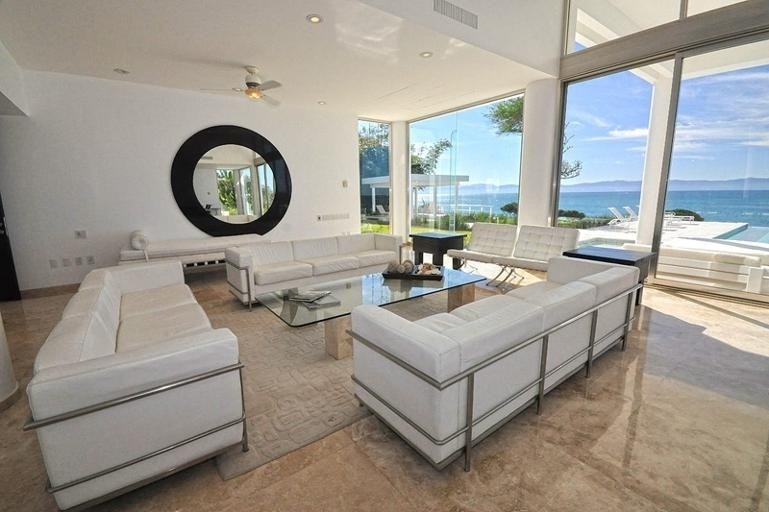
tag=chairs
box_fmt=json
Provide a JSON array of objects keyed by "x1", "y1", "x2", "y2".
[
  {"x1": 376, "y1": 204, "x2": 389, "y2": 215},
  {"x1": 607, "y1": 205, "x2": 694, "y2": 231}
]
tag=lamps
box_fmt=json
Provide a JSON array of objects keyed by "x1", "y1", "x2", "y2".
[{"x1": 244, "y1": 88, "x2": 262, "y2": 102}]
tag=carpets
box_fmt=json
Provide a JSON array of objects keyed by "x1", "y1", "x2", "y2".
[{"x1": 199, "y1": 267, "x2": 525, "y2": 480}]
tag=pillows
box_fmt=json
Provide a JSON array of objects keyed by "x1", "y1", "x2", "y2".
[{"x1": 131, "y1": 230, "x2": 148, "y2": 250}]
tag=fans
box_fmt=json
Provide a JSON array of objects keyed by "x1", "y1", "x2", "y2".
[{"x1": 199, "y1": 65, "x2": 282, "y2": 107}]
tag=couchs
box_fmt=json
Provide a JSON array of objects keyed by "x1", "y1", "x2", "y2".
[
  {"x1": 22, "y1": 257, "x2": 249, "y2": 512},
  {"x1": 117, "y1": 232, "x2": 273, "y2": 276},
  {"x1": 345, "y1": 258, "x2": 644, "y2": 473},
  {"x1": 212, "y1": 214, "x2": 258, "y2": 224}
]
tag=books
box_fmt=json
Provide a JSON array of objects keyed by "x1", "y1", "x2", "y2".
[
  {"x1": 301, "y1": 294, "x2": 341, "y2": 309},
  {"x1": 289, "y1": 290, "x2": 331, "y2": 303}
]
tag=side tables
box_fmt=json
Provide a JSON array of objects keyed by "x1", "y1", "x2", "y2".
[
  {"x1": 561, "y1": 245, "x2": 656, "y2": 306},
  {"x1": 409, "y1": 232, "x2": 466, "y2": 270}
]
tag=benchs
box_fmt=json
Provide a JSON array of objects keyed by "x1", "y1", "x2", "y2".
[
  {"x1": 449, "y1": 222, "x2": 519, "y2": 287},
  {"x1": 224, "y1": 232, "x2": 403, "y2": 312},
  {"x1": 495, "y1": 225, "x2": 579, "y2": 293}
]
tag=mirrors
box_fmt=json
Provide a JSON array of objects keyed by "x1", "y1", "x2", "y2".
[{"x1": 170, "y1": 125, "x2": 292, "y2": 237}]
